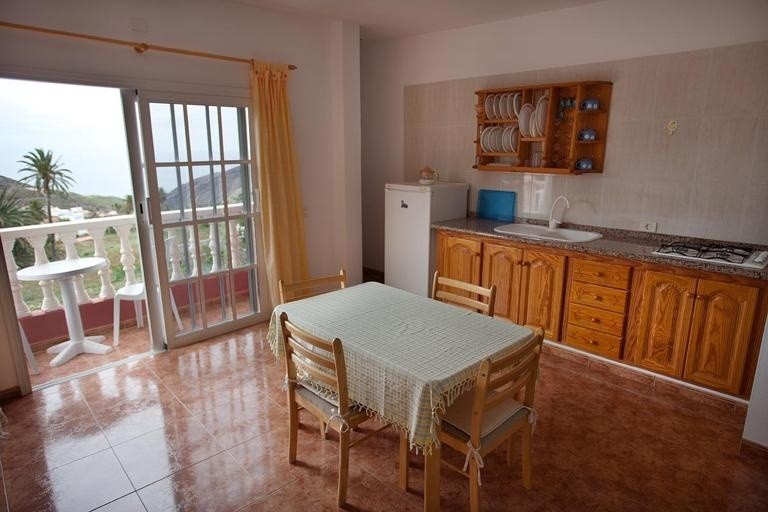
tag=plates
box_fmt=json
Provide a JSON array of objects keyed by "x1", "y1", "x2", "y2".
[
  {"x1": 496, "y1": 127, "x2": 505, "y2": 153},
  {"x1": 518, "y1": 103, "x2": 535, "y2": 137},
  {"x1": 535, "y1": 94, "x2": 549, "y2": 137},
  {"x1": 488, "y1": 126, "x2": 501, "y2": 152},
  {"x1": 499, "y1": 93, "x2": 508, "y2": 119},
  {"x1": 509, "y1": 126, "x2": 519, "y2": 152},
  {"x1": 528, "y1": 109, "x2": 538, "y2": 137},
  {"x1": 484, "y1": 94, "x2": 495, "y2": 120},
  {"x1": 506, "y1": 92, "x2": 515, "y2": 119},
  {"x1": 513, "y1": 92, "x2": 521, "y2": 118},
  {"x1": 501, "y1": 126, "x2": 512, "y2": 152},
  {"x1": 493, "y1": 93, "x2": 501, "y2": 119},
  {"x1": 480, "y1": 125, "x2": 493, "y2": 152}
]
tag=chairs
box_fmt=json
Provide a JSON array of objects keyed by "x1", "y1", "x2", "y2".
[
  {"x1": 431, "y1": 270, "x2": 497, "y2": 317},
  {"x1": 278, "y1": 269, "x2": 348, "y2": 304},
  {"x1": 279, "y1": 311, "x2": 380, "y2": 507},
  {"x1": 112, "y1": 236, "x2": 185, "y2": 345},
  {"x1": 399, "y1": 327, "x2": 545, "y2": 512}
]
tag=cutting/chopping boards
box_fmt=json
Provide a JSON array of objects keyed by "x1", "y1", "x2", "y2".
[{"x1": 477, "y1": 190, "x2": 516, "y2": 222}]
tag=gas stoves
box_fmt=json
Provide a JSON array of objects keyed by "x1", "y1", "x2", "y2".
[{"x1": 651, "y1": 241, "x2": 768, "y2": 269}]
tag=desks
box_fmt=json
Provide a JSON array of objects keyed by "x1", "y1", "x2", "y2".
[
  {"x1": 16, "y1": 257, "x2": 112, "y2": 366},
  {"x1": 266, "y1": 281, "x2": 533, "y2": 492}
]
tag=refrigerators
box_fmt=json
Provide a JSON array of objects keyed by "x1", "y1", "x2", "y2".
[{"x1": 381, "y1": 179, "x2": 468, "y2": 298}]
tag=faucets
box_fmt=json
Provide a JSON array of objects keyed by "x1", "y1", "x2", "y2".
[{"x1": 549, "y1": 196, "x2": 570, "y2": 229}]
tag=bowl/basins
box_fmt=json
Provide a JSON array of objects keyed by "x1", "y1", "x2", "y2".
[
  {"x1": 577, "y1": 127, "x2": 599, "y2": 141},
  {"x1": 580, "y1": 99, "x2": 601, "y2": 110},
  {"x1": 576, "y1": 158, "x2": 594, "y2": 171}
]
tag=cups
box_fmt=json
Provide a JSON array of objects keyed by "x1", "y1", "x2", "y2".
[{"x1": 529, "y1": 150, "x2": 541, "y2": 168}]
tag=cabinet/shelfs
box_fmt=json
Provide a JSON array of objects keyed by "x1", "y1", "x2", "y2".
[
  {"x1": 482, "y1": 233, "x2": 569, "y2": 342},
  {"x1": 561, "y1": 243, "x2": 634, "y2": 362},
  {"x1": 475, "y1": 81, "x2": 613, "y2": 175},
  {"x1": 434, "y1": 224, "x2": 482, "y2": 312},
  {"x1": 623, "y1": 254, "x2": 768, "y2": 401}
]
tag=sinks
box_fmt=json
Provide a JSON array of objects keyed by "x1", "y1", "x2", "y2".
[{"x1": 494, "y1": 223, "x2": 603, "y2": 243}]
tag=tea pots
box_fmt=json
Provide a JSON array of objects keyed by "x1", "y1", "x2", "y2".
[{"x1": 417, "y1": 166, "x2": 441, "y2": 186}]
